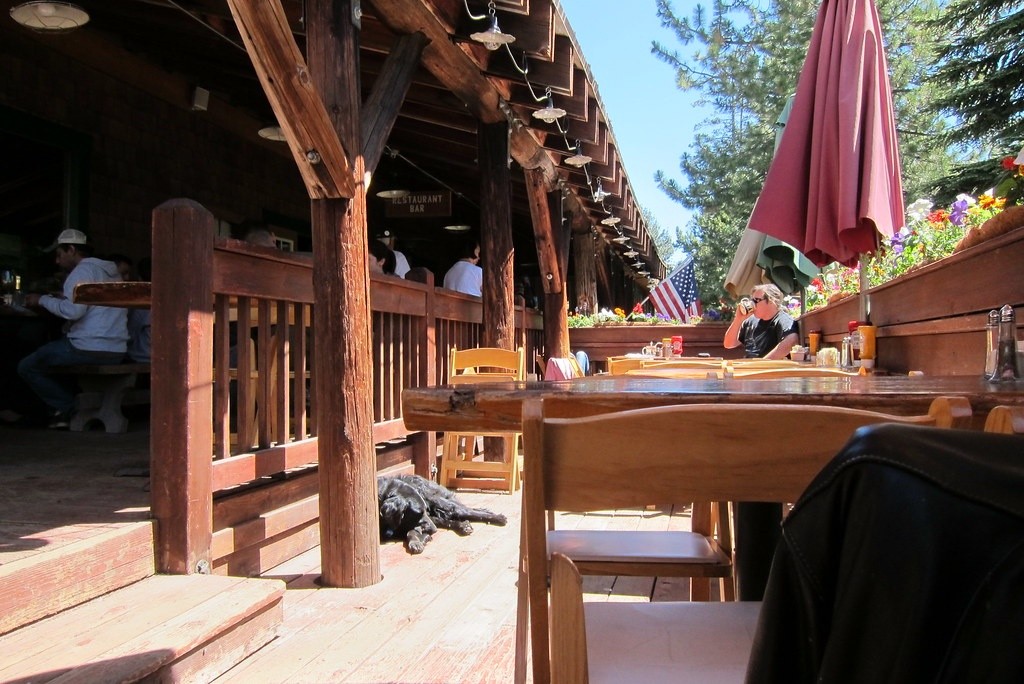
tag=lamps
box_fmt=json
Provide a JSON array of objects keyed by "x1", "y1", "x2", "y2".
[
  {"x1": 631, "y1": 261, "x2": 646, "y2": 269},
  {"x1": 637, "y1": 270, "x2": 651, "y2": 277},
  {"x1": 587, "y1": 185, "x2": 612, "y2": 202},
  {"x1": 470, "y1": 12, "x2": 516, "y2": 51},
  {"x1": 8, "y1": 0, "x2": 91, "y2": 37},
  {"x1": 601, "y1": 212, "x2": 622, "y2": 227},
  {"x1": 612, "y1": 232, "x2": 630, "y2": 245},
  {"x1": 532, "y1": 97, "x2": 566, "y2": 124},
  {"x1": 442, "y1": 214, "x2": 473, "y2": 232},
  {"x1": 563, "y1": 143, "x2": 593, "y2": 169},
  {"x1": 258, "y1": 110, "x2": 287, "y2": 141},
  {"x1": 623, "y1": 249, "x2": 639, "y2": 259},
  {"x1": 374, "y1": 171, "x2": 410, "y2": 199}
]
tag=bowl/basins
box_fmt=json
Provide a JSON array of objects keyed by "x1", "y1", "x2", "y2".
[{"x1": 790, "y1": 351, "x2": 805, "y2": 361}]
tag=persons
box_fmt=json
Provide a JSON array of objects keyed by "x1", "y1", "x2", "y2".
[
  {"x1": 0, "y1": 221, "x2": 539, "y2": 431},
  {"x1": 723, "y1": 284, "x2": 799, "y2": 359}
]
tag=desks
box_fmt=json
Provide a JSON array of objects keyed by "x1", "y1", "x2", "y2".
[{"x1": 404, "y1": 377, "x2": 1024, "y2": 602}]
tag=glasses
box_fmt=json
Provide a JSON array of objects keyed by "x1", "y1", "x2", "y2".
[{"x1": 752, "y1": 298, "x2": 767, "y2": 304}]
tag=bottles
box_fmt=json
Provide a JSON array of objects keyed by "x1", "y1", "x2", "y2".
[
  {"x1": 988, "y1": 304, "x2": 1024, "y2": 383},
  {"x1": 657, "y1": 336, "x2": 682, "y2": 358},
  {"x1": 983, "y1": 309, "x2": 1001, "y2": 380},
  {"x1": 808, "y1": 320, "x2": 877, "y2": 368}
]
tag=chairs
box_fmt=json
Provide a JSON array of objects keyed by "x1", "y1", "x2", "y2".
[{"x1": 436, "y1": 342, "x2": 1024, "y2": 684}]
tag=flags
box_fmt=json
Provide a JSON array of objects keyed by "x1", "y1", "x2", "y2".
[{"x1": 648, "y1": 256, "x2": 701, "y2": 324}]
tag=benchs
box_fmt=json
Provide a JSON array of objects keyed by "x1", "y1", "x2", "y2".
[{"x1": 67, "y1": 363, "x2": 142, "y2": 435}]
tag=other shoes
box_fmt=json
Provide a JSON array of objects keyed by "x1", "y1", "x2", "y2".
[{"x1": 47, "y1": 410, "x2": 70, "y2": 428}]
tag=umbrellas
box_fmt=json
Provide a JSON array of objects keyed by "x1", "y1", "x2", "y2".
[{"x1": 723, "y1": 0, "x2": 904, "y2": 347}]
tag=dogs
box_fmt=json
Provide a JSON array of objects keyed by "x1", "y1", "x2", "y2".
[{"x1": 376, "y1": 474, "x2": 506, "y2": 555}]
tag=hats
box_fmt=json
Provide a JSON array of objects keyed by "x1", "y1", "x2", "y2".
[
  {"x1": 48, "y1": 229, "x2": 89, "y2": 252},
  {"x1": 375, "y1": 226, "x2": 393, "y2": 238}
]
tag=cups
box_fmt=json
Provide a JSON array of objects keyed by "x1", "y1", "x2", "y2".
[
  {"x1": 740, "y1": 298, "x2": 756, "y2": 316},
  {"x1": 642, "y1": 341, "x2": 656, "y2": 356}
]
